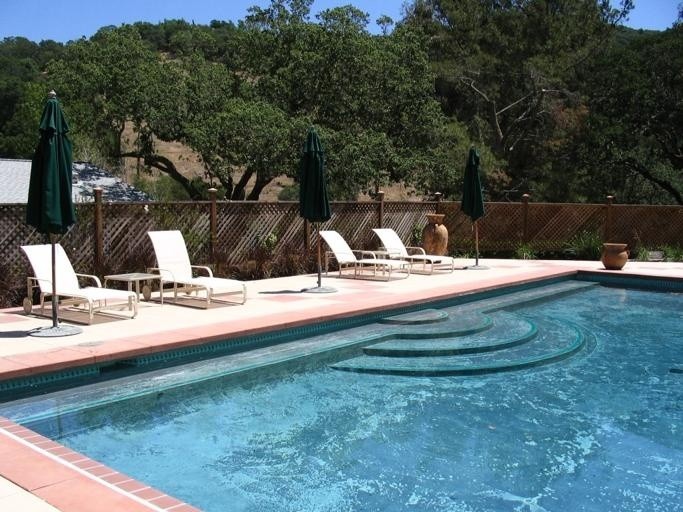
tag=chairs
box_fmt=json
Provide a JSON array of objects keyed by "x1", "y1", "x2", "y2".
[
  {"x1": 21, "y1": 229, "x2": 248, "y2": 325},
  {"x1": 317, "y1": 223, "x2": 457, "y2": 280}
]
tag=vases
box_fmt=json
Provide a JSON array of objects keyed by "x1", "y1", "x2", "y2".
[
  {"x1": 600, "y1": 243, "x2": 629, "y2": 268},
  {"x1": 423, "y1": 214, "x2": 449, "y2": 256}
]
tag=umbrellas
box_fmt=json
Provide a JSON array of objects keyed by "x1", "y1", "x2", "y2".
[
  {"x1": 460, "y1": 145, "x2": 485, "y2": 265},
  {"x1": 26, "y1": 91, "x2": 77, "y2": 328},
  {"x1": 298, "y1": 126, "x2": 331, "y2": 287}
]
